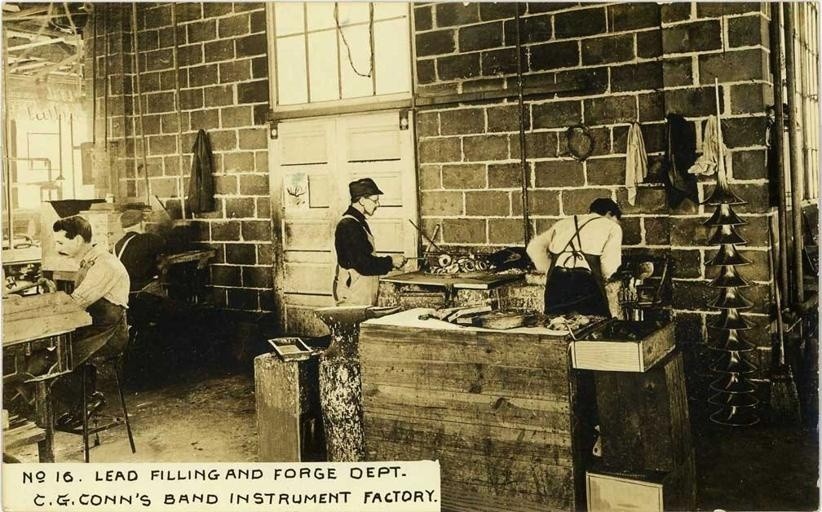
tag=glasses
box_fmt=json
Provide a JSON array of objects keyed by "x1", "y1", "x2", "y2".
[{"x1": 367, "y1": 198, "x2": 378, "y2": 204}]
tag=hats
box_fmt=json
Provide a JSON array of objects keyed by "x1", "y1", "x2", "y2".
[
  {"x1": 121, "y1": 207, "x2": 145, "y2": 227},
  {"x1": 348, "y1": 178, "x2": 381, "y2": 203}
]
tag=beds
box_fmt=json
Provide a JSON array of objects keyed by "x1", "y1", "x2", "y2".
[{"x1": 2, "y1": 291, "x2": 93, "y2": 463}]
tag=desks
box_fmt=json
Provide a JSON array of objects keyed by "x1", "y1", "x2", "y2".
[{"x1": 359, "y1": 308, "x2": 618, "y2": 512}]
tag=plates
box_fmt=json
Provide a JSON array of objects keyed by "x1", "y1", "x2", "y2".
[{"x1": 267, "y1": 337, "x2": 314, "y2": 362}]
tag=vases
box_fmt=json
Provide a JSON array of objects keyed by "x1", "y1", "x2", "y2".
[{"x1": 313, "y1": 305, "x2": 376, "y2": 462}]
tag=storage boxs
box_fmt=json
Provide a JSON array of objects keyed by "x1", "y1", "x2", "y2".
[{"x1": 570, "y1": 320, "x2": 676, "y2": 374}]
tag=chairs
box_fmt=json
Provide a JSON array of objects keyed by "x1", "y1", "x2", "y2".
[{"x1": 53, "y1": 325, "x2": 139, "y2": 463}]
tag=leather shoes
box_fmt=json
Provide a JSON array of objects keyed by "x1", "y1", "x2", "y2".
[
  {"x1": 57, "y1": 406, "x2": 75, "y2": 426},
  {"x1": 67, "y1": 392, "x2": 106, "y2": 427}
]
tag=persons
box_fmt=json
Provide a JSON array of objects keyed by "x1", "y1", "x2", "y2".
[
  {"x1": 526, "y1": 198, "x2": 623, "y2": 319},
  {"x1": 23, "y1": 214, "x2": 131, "y2": 428},
  {"x1": 115, "y1": 209, "x2": 216, "y2": 355},
  {"x1": 332, "y1": 178, "x2": 407, "y2": 307}
]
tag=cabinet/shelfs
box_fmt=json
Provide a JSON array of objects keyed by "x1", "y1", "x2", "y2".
[
  {"x1": 594, "y1": 349, "x2": 693, "y2": 482},
  {"x1": 40, "y1": 201, "x2": 152, "y2": 271}
]
